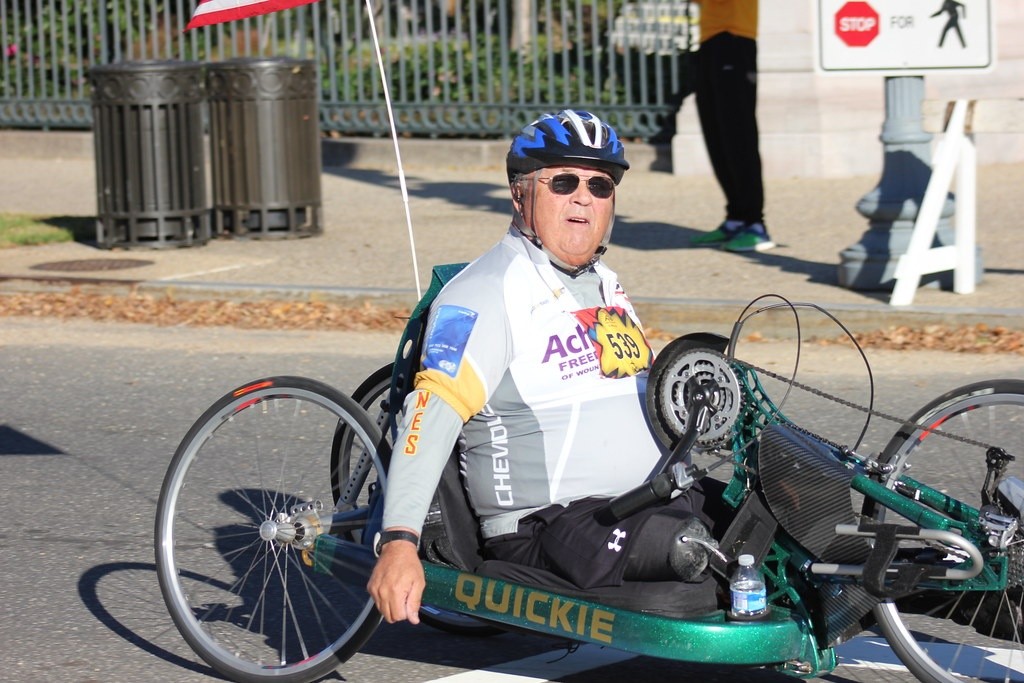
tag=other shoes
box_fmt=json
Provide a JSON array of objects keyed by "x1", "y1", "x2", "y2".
[
  {"x1": 684, "y1": 218, "x2": 745, "y2": 244},
  {"x1": 718, "y1": 221, "x2": 774, "y2": 250}
]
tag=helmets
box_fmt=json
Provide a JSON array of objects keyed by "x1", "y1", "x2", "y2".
[{"x1": 506, "y1": 108, "x2": 630, "y2": 185}]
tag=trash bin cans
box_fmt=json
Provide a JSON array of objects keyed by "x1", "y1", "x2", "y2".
[
  {"x1": 84, "y1": 59, "x2": 211, "y2": 252},
  {"x1": 202, "y1": 57, "x2": 324, "y2": 244}
]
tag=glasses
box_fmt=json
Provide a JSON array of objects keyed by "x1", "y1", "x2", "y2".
[{"x1": 517, "y1": 173, "x2": 616, "y2": 199}]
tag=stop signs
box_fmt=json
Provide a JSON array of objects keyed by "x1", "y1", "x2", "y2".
[{"x1": 834, "y1": 1, "x2": 879, "y2": 48}]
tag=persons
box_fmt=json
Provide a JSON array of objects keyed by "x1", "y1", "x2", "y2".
[
  {"x1": 687, "y1": 0, "x2": 776, "y2": 253},
  {"x1": 365, "y1": 105, "x2": 892, "y2": 648}
]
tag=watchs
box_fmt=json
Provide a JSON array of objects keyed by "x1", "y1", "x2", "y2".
[{"x1": 373, "y1": 530, "x2": 421, "y2": 558}]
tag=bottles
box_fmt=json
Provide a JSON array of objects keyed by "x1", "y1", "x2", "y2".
[{"x1": 729, "y1": 553, "x2": 767, "y2": 620}]
